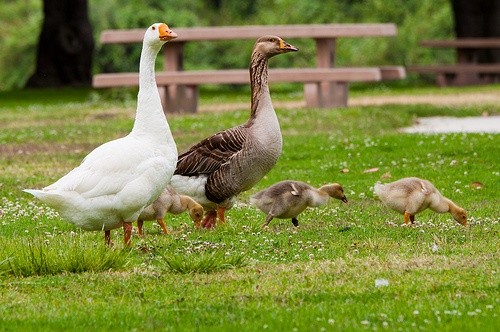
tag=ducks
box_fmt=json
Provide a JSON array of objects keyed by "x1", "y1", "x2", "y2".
[
  {"x1": 21, "y1": 22, "x2": 178, "y2": 247},
  {"x1": 138, "y1": 187, "x2": 204, "y2": 236},
  {"x1": 248, "y1": 182, "x2": 348, "y2": 227},
  {"x1": 176, "y1": 36, "x2": 300, "y2": 228},
  {"x1": 373, "y1": 177, "x2": 468, "y2": 224}
]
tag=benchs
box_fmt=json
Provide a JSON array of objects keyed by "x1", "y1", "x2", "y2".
[
  {"x1": 407, "y1": 62, "x2": 500, "y2": 86},
  {"x1": 90, "y1": 64, "x2": 384, "y2": 118}
]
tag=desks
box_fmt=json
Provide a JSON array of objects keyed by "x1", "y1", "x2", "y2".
[
  {"x1": 98, "y1": 21, "x2": 397, "y2": 113},
  {"x1": 419, "y1": 37, "x2": 499, "y2": 64}
]
